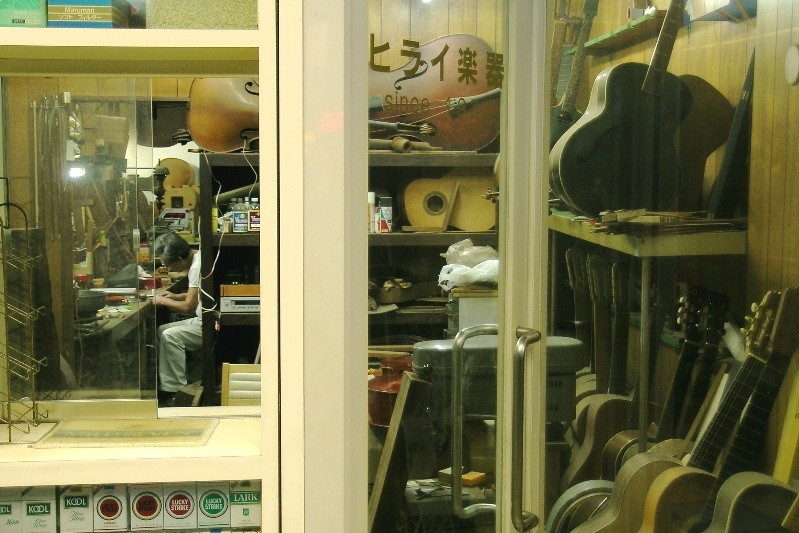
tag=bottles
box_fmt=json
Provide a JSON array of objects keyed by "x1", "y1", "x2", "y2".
[
  {"x1": 140, "y1": 243, "x2": 149, "y2": 263},
  {"x1": 231, "y1": 197, "x2": 260, "y2": 235}
]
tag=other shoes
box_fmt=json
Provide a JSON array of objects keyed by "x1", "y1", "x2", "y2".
[{"x1": 157, "y1": 390, "x2": 178, "y2": 403}]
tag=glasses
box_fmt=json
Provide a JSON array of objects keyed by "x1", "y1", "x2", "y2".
[{"x1": 162, "y1": 264, "x2": 170, "y2": 269}]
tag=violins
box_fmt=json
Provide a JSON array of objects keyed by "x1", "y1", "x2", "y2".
[
  {"x1": 169, "y1": 32, "x2": 501, "y2": 151},
  {"x1": 368, "y1": 356, "x2": 414, "y2": 428},
  {"x1": 186, "y1": 78, "x2": 436, "y2": 154}
]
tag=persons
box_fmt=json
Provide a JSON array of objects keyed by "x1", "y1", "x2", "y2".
[{"x1": 151, "y1": 232, "x2": 203, "y2": 402}]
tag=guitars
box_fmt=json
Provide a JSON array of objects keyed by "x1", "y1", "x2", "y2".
[
  {"x1": 551, "y1": 0, "x2": 572, "y2": 106},
  {"x1": 549, "y1": 0, "x2": 693, "y2": 219},
  {"x1": 550, "y1": 0, "x2": 599, "y2": 154},
  {"x1": 547, "y1": 249, "x2": 798, "y2": 533},
  {"x1": 404, "y1": 167, "x2": 497, "y2": 233}
]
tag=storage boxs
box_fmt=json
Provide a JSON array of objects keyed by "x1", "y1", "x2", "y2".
[{"x1": 0, "y1": 0, "x2": 130, "y2": 29}]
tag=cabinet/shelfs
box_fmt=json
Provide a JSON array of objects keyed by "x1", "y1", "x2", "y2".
[{"x1": 197, "y1": 147, "x2": 499, "y2": 409}]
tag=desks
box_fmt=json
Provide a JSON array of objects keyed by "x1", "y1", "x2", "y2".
[
  {"x1": 545, "y1": 206, "x2": 750, "y2": 455},
  {"x1": 75, "y1": 267, "x2": 188, "y2": 390}
]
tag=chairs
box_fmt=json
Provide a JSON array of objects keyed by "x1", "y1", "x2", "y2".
[{"x1": 221, "y1": 362, "x2": 261, "y2": 408}]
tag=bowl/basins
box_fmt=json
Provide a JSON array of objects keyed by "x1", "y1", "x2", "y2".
[{"x1": 74, "y1": 289, "x2": 106, "y2": 313}]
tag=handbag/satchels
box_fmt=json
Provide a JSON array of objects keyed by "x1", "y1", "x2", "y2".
[
  {"x1": 438, "y1": 259, "x2": 499, "y2": 291},
  {"x1": 440, "y1": 238, "x2": 497, "y2": 268}
]
